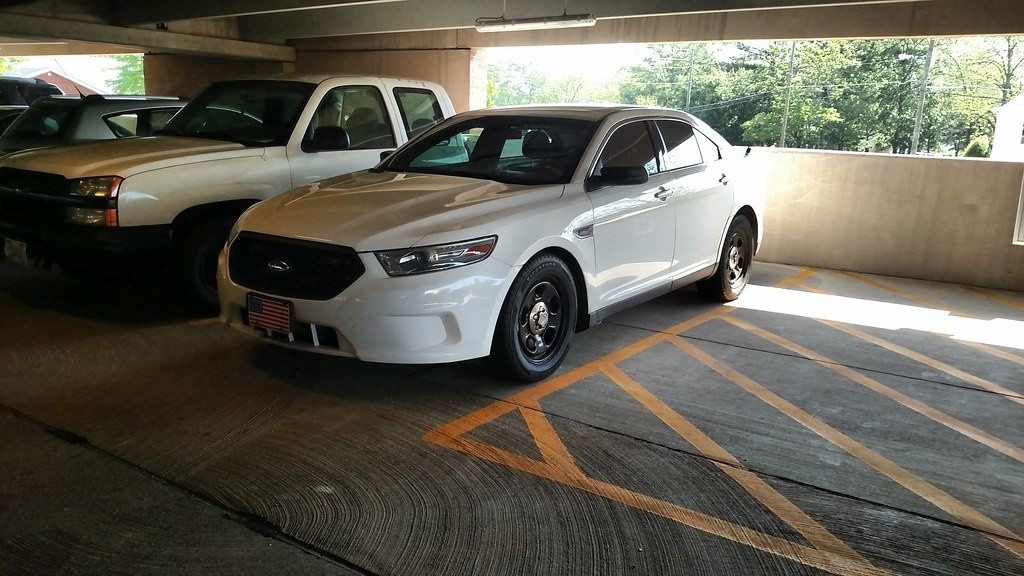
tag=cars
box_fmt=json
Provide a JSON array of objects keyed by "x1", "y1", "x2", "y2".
[
  {"x1": 0, "y1": 96, "x2": 263, "y2": 178},
  {"x1": 217, "y1": 103, "x2": 769, "y2": 382},
  {"x1": 0, "y1": 106, "x2": 137, "y2": 140}
]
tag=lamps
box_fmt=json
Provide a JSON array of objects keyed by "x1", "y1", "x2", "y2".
[{"x1": 474, "y1": 0, "x2": 598, "y2": 34}]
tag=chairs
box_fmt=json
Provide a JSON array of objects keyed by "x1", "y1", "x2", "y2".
[
  {"x1": 345, "y1": 107, "x2": 378, "y2": 134},
  {"x1": 522, "y1": 131, "x2": 549, "y2": 171},
  {"x1": 411, "y1": 118, "x2": 435, "y2": 136}
]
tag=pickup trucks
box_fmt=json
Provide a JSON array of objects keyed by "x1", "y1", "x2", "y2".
[{"x1": 0, "y1": 77, "x2": 524, "y2": 315}]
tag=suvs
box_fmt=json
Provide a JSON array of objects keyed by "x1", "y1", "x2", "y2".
[{"x1": 0, "y1": 76, "x2": 67, "y2": 104}]
